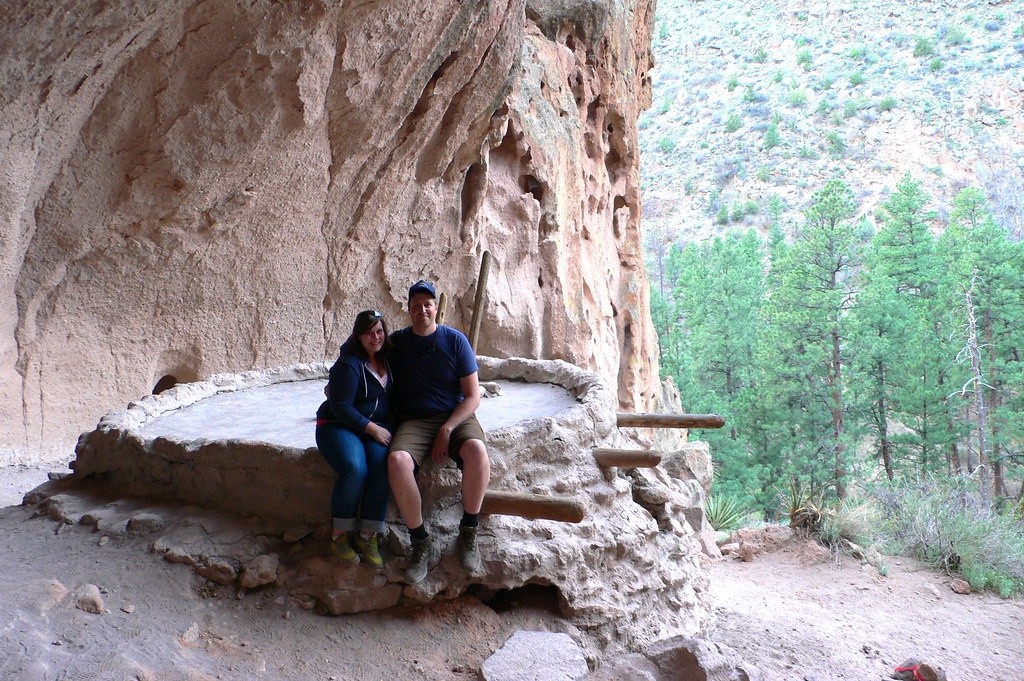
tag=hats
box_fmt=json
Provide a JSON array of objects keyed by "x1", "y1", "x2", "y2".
[{"x1": 409, "y1": 280, "x2": 436, "y2": 299}]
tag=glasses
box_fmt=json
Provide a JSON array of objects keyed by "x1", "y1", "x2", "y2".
[
  {"x1": 409, "y1": 336, "x2": 436, "y2": 361},
  {"x1": 358, "y1": 310, "x2": 381, "y2": 319}
]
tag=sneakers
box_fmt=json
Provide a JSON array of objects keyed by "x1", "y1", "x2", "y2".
[
  {"x1": 457, "y1": 519, "x2": 480, "y2": 571},
  {"x1": 352, "y1": 532, "x2": 383, "y2": 569},
  {"x1": 329, "y1": 531, "x2": 360, "y2": 568},
  {"x1": 404, "y1": 534, "x2": 436, "y2": 583}
]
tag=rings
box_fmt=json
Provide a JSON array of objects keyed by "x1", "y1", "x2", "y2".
[{"x1": 443, "y1": 452, "x2": 447, "y2": 457}]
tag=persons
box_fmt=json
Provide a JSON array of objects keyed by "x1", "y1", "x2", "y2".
[
  {"x1": 315, "y1": 310, "x2": 394, "y2": 569},
  {"x1": 323, "y1": 280, "x2": 491, "y2": 583}
]
